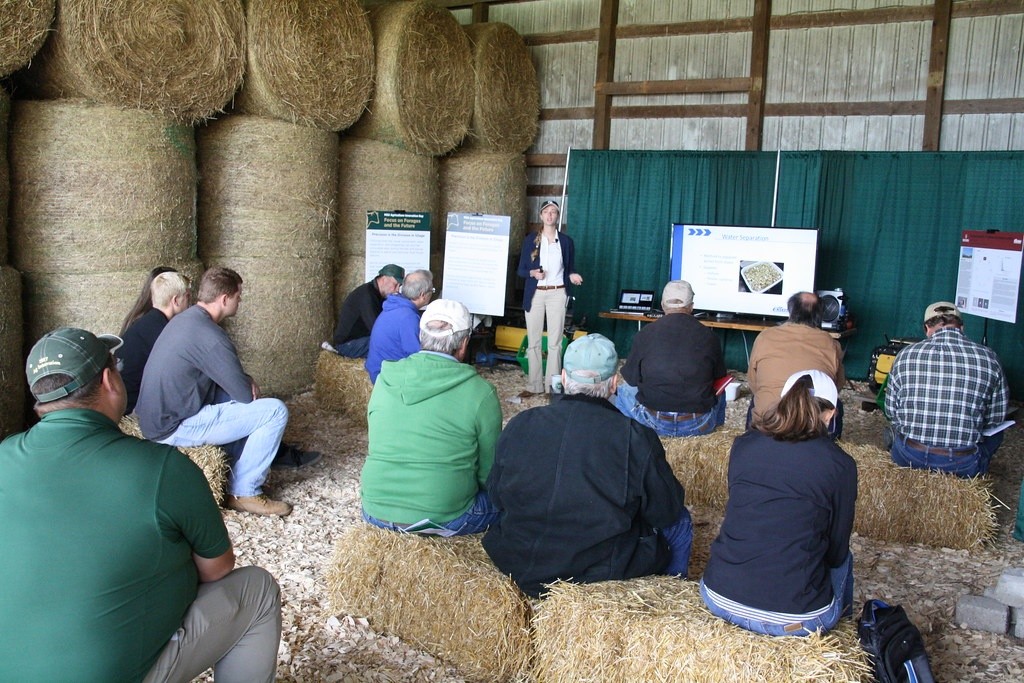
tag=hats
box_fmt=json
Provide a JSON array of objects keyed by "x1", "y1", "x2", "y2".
[
  {"x1": 418, "y1": 298, "x2": 472, "y2": 338},
  {"x1": 924, "y1": 302, "x2": 964, "y2": 333},
  {"x1": 378, "y1": 264, "x2": 404, "y2": 286},
  {"x1": 780, "y1": 371, "x2": 837, "y2": 434},
  {"x1": 25, "y1": 327, "x2": 124, "y2": 404},
  {"x1": 563, "y1": 334, "x2": 618, "y2": 384},
  {"x1": 540, "y1": 201, "x2": 561, "y2": 214},
  {"x1": 662, "y1": 282, "x2": 695, "y2": 309}
]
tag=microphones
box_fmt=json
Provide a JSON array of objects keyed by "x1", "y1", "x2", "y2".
[{"x1": 555, "y1": 239, "x2": 558, "y2": 242}]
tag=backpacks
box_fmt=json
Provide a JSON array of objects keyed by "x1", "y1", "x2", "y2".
[{"x1": 857, "y1": 598, "x2": 933, "y2": 683}]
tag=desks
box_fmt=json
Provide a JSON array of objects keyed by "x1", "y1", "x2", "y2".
[{"x1": 599, "y1": 311, "x2": 858, "y2": 368}]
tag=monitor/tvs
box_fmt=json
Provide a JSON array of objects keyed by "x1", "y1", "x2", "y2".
[{"x1": 669, "y1": 223, "x2": 820, "y2": 321}]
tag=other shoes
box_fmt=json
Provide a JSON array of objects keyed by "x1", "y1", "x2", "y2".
[
  {"x1": 517, "y1": 389, "x2": 537, "y2": 399},
  {"x1": 273, "y1": 450, "x2": 324, "y2": 468}
]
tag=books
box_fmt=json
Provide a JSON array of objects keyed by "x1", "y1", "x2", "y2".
[
  {"x1": 713, "y1": 375, "x2": 735, "y2": 395},
  {"x1": 983, "y1": 420, "x2": 1016, "y2": 436}
]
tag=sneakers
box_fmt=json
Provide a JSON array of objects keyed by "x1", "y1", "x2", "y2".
[{"x1": 224, "y1": 493, "x2": 290, "y2": 518}]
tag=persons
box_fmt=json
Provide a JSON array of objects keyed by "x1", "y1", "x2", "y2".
[
  {"x1": 884, "y1": 301, "x2": 1009, "y2": 479},
  {"x1": 745, "y1": 292, "x2": 844, "y2": 439},
  {"x1": 112, "y1": 272, "x2": 323, "y2": 472},
  {"x1": 359, "y1": 299, "x2": 503, "y2": 537},
  {"x1": 119, "y1": 266, "x2": 178, "y2": 335},
  {"x1": 516, "y1": 201, "x2": 583, "y2": 400},
  {"x1": 137, "y1": 266, "x2": 292, "y2": 517},
  {"x1": 479, "y1": 334, "x2": 693, "y2": 601},
  {"x1": 365, "y1": 269, "x2": 435, "y2": 386},
  {"x1": 614, "y1": 280, "x2": 726, "y2": 439},
  {"x1": 334, "y1": 264, "x2": 405, "y2": 359},
  {"x1": 0, "y1": 328, "x2": 281, "y2": 683},
  {"x1": 700, "y1": 369, "x2": 858, "y2": 638}
]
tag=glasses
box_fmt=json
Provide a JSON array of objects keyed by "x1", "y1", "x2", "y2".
[{"x1": 422, "y1": 287, "x2": 436, "y2": 294}]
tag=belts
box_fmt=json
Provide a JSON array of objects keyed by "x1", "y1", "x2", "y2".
[
  {"x1": 898, "y1": 432, "x2": 977, "y2": 456},
  {"x1": 536, "y1": 284, "x2": 565, "y2": 290},
  {"x1": 648, "y1": 407, "x2": 701, "y2": 422}
]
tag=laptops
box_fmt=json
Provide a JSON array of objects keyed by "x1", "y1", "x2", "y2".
[{"x1": 611, "y1": 289, "x2": 656, "y2": 316}]
